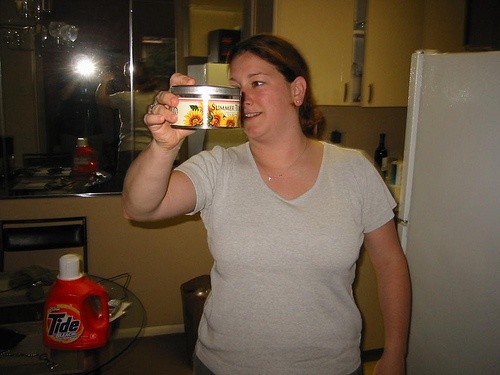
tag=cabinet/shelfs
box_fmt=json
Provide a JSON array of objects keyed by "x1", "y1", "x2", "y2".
[{"x1": 254, "y1": 0, "x2": 466, "y2": 108}]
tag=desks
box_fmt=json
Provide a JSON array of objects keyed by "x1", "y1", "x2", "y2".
[
  {"x1": 0, "y1": 165, "x2": 113, "y2": 194},
  {"x1": 0, "y1": 269, "x2": 146, "y2": 375}
]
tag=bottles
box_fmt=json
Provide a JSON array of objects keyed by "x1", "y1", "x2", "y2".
[
  {"x1": 42, "y1": 252, "x2": 109, "y2": 351},
  {"x1": 375, "y1": 133, "x2": 388, "y2": 182},
  {"x1": 71, "y1": 137, "x2": 98, "y2": 174}
]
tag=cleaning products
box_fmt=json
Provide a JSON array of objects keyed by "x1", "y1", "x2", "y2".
[
  {"x1": 72, "y1": 138, "x2": 98, "y2": 174},
  {"x1": 43, "y1": 253, "x2": 109, "y2": 350}
]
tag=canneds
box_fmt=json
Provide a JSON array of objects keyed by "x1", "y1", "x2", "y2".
[{"x1": 170, "y1": 85, "x2": 243, "y2": 129}]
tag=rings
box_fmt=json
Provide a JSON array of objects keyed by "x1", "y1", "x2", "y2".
[{"x1": 148, "y1": 102, "x2": 159, "y2": 114}]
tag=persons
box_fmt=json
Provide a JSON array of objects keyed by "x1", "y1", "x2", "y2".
[
  {"x1": 120, "y1": 33, "x2": 413, "y2": 375},
  {"x1": 94, "y1": 60, "x2": 162, "y2": 193}
]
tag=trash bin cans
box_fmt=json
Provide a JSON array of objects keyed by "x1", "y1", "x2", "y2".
[{"x1": 181, "y1": 274, "x2": 211, "y2": 368}]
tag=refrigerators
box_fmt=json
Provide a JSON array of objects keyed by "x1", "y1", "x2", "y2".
[
  {"x1": 188, "y1": 63, "x2": 249, "y2": 156},
  {"x1": 397, "y1": 51, "x2": 500, "y2": 375}
]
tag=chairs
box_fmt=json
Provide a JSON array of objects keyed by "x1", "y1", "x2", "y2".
[
  {"x1": 0, "y1": 217, "x2": 89, "y2": 272},
  {"x1": 22, "y1": 149, "x2": 74, "y2": 169}
]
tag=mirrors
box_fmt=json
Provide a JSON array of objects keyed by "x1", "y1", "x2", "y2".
[{"x1": 0, "y1": 0, "x2": 274, "y2": 202}]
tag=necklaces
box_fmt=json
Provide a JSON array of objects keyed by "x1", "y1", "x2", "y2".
[{"x1": 252, "y1": 136, "x2": 310, "y2": 182}]
